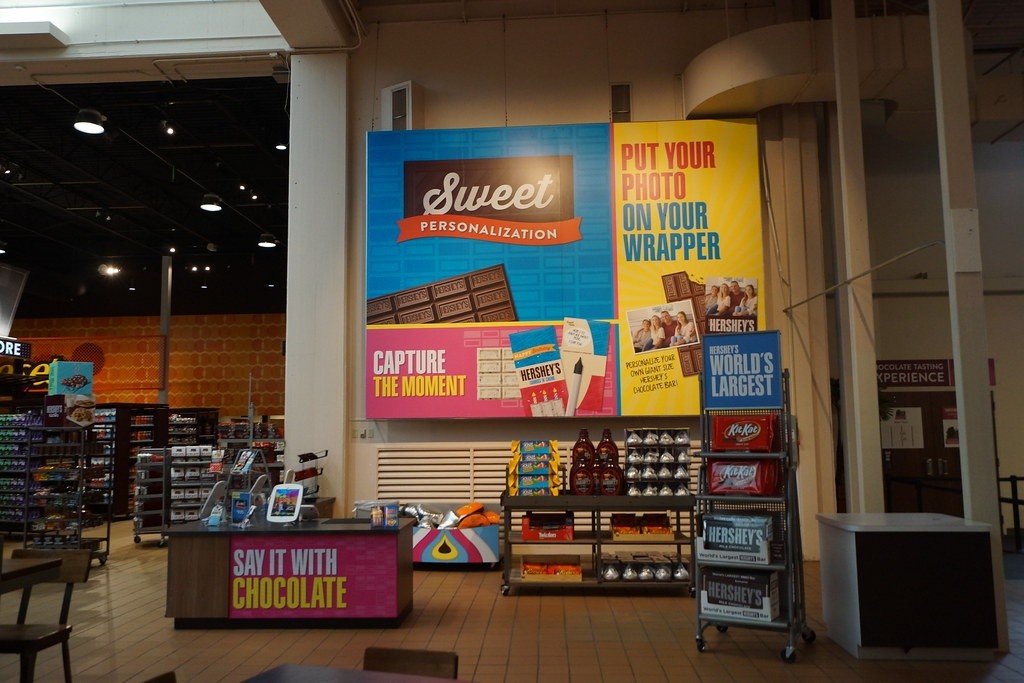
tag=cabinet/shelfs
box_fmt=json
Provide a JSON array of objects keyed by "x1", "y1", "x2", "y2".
[
  {"x1": 23, "y1": 425, "x2": 113, "y2": 567},
  {"x1": 499, "y1": 489, "x2": 697, "y2": 596},
  {"x1": 0, "y1": 402, "x2": 46, "y2": 542},
  {"x1": 82, "y1": 406, "x2": 126, "y2": 520},
  {"x1": 695, "y1": 372, "x2": 814, "y2": 663},
  {"x1": 132, "y1": 446, "x2": 224, "y2": 547},
  {"x1": 128, "y1": 407, "x2": 169, "y2": 516},
  {"x1": 168, "y1": 412, "x2": 202, "y2": 447}
]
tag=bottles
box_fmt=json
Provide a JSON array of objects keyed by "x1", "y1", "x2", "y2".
[{"x1": 569, "y1": 428, "x2": 623, "y2": 496}]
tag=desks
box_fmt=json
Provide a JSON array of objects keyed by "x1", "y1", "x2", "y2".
[
  {"x1": 815, "y1": 513, "x2": 1000, "y2": 661},
  {"x1": 0, "y1": 556, "x2": 62, "y2": 596},
  {"x1": 239, "y1": 663, "x2": 477, "y2": 683}
]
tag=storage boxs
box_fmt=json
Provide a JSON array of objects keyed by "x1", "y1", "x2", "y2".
[
  {"x1": 696, "y1": 513, "x2": 773, "y2": 564},
  {"x1": 48, "y1": 361, "x2": 93, "y2": 395},
  {"x1": 512, "y1": 440, "x2": 557, "y2": 453},
  {"x1": 659, "y1": 428, "x2": 690, "y2": 447},
  {"x1": 509, "y1": 487, "x2": 559, "y2": 497},
  {"x1": 701, "y1": 566, "x2": 779, "y2": 622},
  {"x1": 510, "y1": 460, "x2": 557, "y2": 475},
  {"x1": 514, "y1": 453, "x2": 560, "y2": 464},
  {"x1": 714, "y1": 414, "x2": 773, "y2": 450},
  {"x1": 520, "y1": 556, "x2": 582, "y2": 582},
  {"x1": 609, "y1": 513, "x2": 675, "y2": 542},
  {"x1": 171, "y1": 445, "x2": 214, "y2": 522},
  {"x1": 624, "y1": 428, "x2": 659, "y2": 447},
  {"x1": 508, "y1": 475, "x2": 561, "y2": 488},
  {"x1": 45, "y1": 395, "x2": 95, "y2": 426},
  {"x1": 625, "y1": 447, "x2": 691, "y2": 496},
  {"x1": 522, "y1": 510, "x2": 574, "y2": 540}
]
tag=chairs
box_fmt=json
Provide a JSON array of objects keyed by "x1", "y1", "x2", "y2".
[
  {"x1": 363, "y1": 647, "x2": 459, "y2": 680},
  {"x1": 0, "y1": 549, "x2": 94, "y2": 683}
]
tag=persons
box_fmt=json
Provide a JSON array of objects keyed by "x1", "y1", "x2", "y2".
[
  {"x1": 632, "y1": 310, "x2": 697, "y2": 353},
  {"x1": 703, "y1": 281, "x2": 758, "y2": 319}
]
